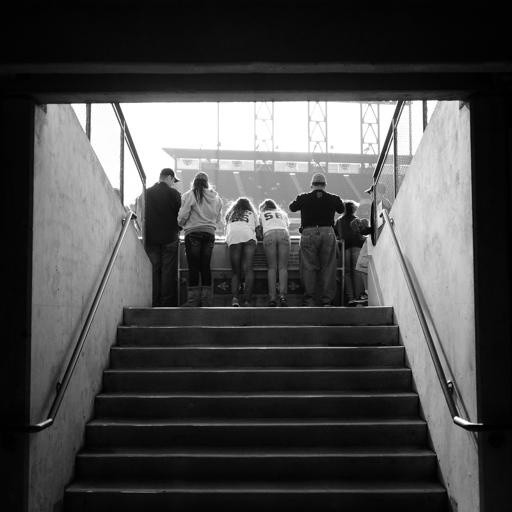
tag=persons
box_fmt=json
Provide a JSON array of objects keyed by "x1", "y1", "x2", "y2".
[
  {"x1": 223, "y1": 196, "x2": 260, "y2": 308},
  {"x1": 349, "y1": 184, "x2": 393, "y2": 305},
  {"x1": 140, "y1": 169, "x2": 183, "y2": 310},
  {"x1": 259, "y1": 198, "x2": 292, "y2": 308},
  {"x1": 289, "y1": 172, "x2": 345, "y2": 306},
  {"x1": 178, "y1": 172, "x2": 223, "y2": 307},
  {"x1": 332, "y1": 199, "x2": 369, "y2": 306}
]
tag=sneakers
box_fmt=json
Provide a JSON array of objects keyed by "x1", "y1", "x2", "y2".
[
  {"x1": 345, "y1": 290, "x2": 369, "y2": 307},
  {"x1": 297, "y1": 301, "x2": 336, "y2": 306},
  {"x1": 231, "y1": 297, "x2": 250, "y2": 306},
  {"x1": 269, "y1": 296, "x2": 288, "y2": 307}
]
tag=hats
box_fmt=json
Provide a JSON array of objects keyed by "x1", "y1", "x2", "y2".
[
  {"x1": 159, "y1": 168, "x2": 180, "y2": 184},
  {"x1": 194, "y1": 172, "x2": 209, "y2": 181},
  {"x1": 311, "y1": 173, "x2": 325, "y2": 183},
  {"x1": 364, "y1": 182, "x2": 386, "y2": 194}
]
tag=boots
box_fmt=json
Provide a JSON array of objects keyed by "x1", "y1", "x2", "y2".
[
  {"x1": 200, "y1": 285, "x2": 212, "y2": 306},
  {"x1": 179, "y1": 286, "x2": 200, "y2": 307}
]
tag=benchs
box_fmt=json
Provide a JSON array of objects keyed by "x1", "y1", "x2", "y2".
[{"x1": 177, "y1": 167, "x2": 405, "y2": 217}]
